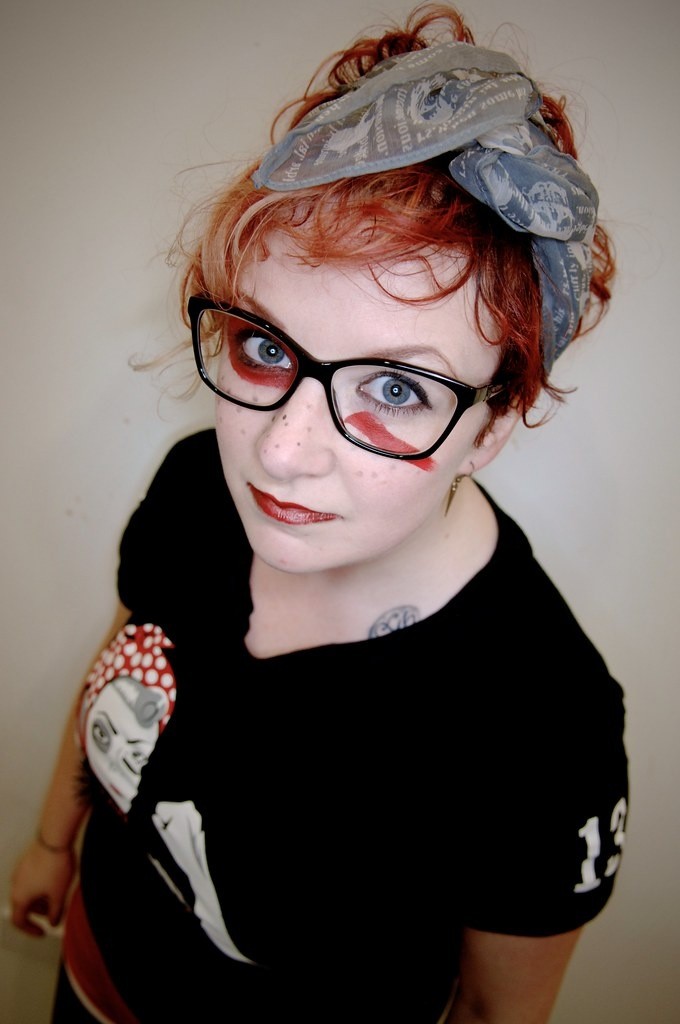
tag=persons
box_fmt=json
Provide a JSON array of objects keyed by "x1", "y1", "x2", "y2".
[{"x1": 10, "y1": 3, "x2": 630, "y2": 1024}]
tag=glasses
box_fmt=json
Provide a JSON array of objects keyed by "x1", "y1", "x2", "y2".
[{"x1": 187, "y1": 289, "x2": 512, "y2": 460}]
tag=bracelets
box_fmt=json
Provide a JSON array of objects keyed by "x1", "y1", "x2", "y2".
[{"x1": 36, "y1": 829, "x2": 73, "y2": 853}]
